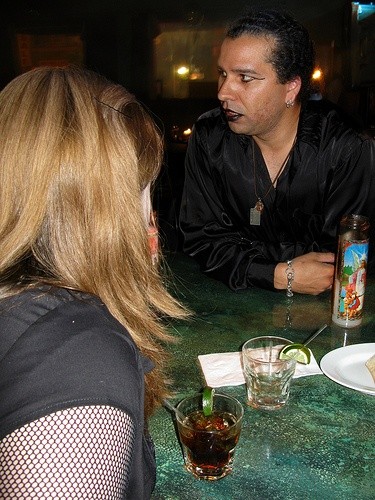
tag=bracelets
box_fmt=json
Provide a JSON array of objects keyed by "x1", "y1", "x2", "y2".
[{"x1": 285, "y1": 260, "x2": 295, "y2": 298}]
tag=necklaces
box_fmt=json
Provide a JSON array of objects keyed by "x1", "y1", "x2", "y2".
[{"x1": 250, "y1": 137, "x2": 299, "y2": 226}]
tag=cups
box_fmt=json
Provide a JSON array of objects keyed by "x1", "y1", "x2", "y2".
[
  {"x1": 242, "y1": 336, "x2": 299, "y2": 409},
  {"x1": 173, "y1": 392, "x2": 245, "y2": 481}
]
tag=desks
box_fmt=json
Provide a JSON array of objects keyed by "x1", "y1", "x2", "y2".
[{"x1": 148, "y1": 123, "x2": 375, "y2": 500}]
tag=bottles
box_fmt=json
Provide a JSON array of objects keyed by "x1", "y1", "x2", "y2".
[{"x1": 332, "y1": 213, "x2": 369, "y2": 328}]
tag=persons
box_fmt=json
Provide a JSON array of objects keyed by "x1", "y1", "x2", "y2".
[
  {"x1": 0, "y1": 65, "x2": 195, "y2": 500},
  {"x1": 179, "y1": 9, "x2": 375, "y2": 295}
]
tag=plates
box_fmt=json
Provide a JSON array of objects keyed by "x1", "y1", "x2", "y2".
[{"x1": 320, "y1": 343, "x2": 375, "y2": 395}]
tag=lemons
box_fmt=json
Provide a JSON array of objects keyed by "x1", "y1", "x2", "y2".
[
  {"x1": 202, "y1": 386, "x2": 215, "y2": 416},
  {"x1": 278, "y1": 343, "x2": 310, "y2": 359},
  {"x1": 283, "y1": 346, "x2": 310, "y2": 365}
]
